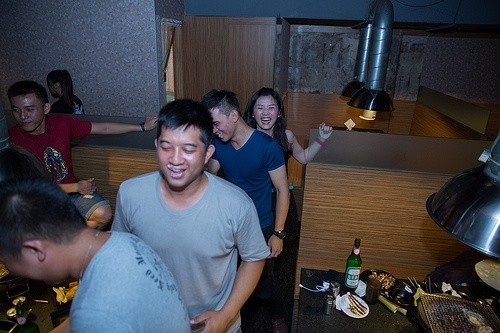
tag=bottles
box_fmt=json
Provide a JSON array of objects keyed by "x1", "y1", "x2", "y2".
[
  {"x1": 345, "y1": 238, "x2": 363, "y2": 289},
  {"x1": 323, "y1": 294, "x2": 333, "y2": 315}
]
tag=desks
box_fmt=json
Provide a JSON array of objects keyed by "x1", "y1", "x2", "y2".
[
  {"x1": 295, "y1": 268, "x2": 500, "y2": 333},
  {"x1": 0, "y1": 274, "x2": 78, "y2": 333}
]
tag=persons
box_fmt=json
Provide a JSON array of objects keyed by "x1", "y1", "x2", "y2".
[
  {"x1": 112, "y1": 99, "x2": 271, "y2": 333},
  {"x1": 202, "y1": 87, "x2": 332, "y2": 333},
  {"x1": 0, "y1": 176, "x2": 191, "y2": 333},
  {"x1": 0, "y1": 70, "x2": 159, "y2": 230}
]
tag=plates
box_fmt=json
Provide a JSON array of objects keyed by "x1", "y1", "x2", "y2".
[
  {"x1": 341, "y1": 295, "x2": 369, "y2": 317},
  {"x1": 359, "y1": 270, "x2": 400, "y2": 292}
]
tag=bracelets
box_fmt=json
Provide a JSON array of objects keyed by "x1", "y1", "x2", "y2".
[
  {"x1": 315, "y1": 137, "x2": 325, "y2": 146},
  {"x1": 139, "y1": 122, "x2": 145, "y2": 131}
]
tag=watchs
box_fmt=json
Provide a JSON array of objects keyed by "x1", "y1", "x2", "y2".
[{"x1": 272, "y1": 230, "x2": 286, "y2": 239}]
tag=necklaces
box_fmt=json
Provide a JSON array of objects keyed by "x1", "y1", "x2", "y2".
[{"x1": 79, "y1": 230, "x2": 100, "y2": 285}]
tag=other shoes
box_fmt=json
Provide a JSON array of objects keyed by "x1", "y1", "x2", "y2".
[{"x1": 272, "y1": 316, "x2": 288, "y2": 333}]
